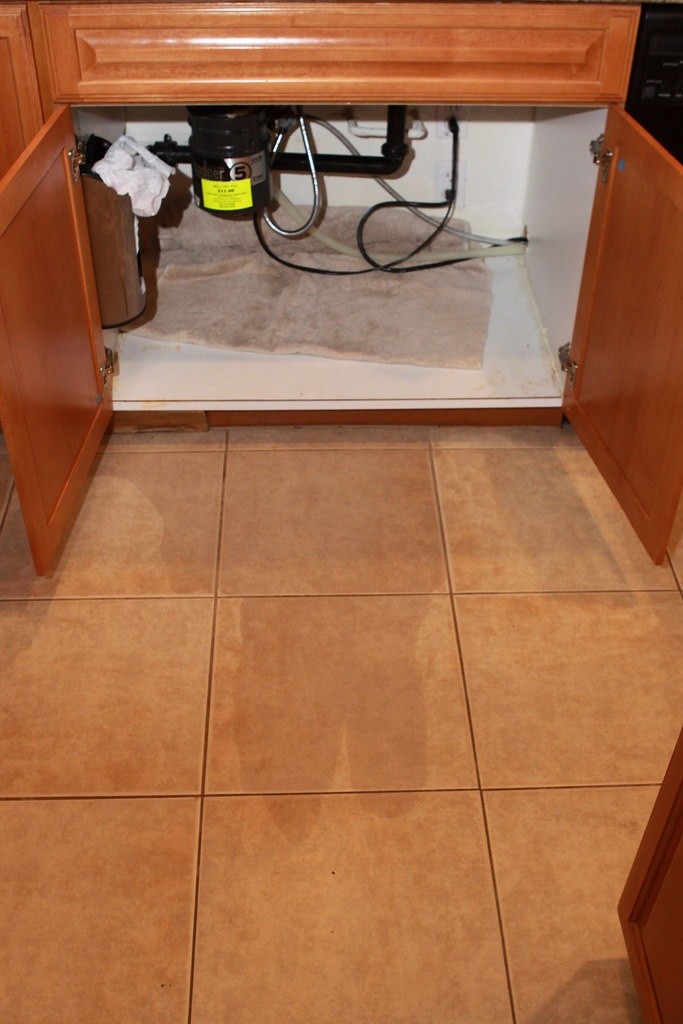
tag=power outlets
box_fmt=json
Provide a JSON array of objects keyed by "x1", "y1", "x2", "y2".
[{"x1": 435, "y1": 106, "x2": 469, "y2": 139}]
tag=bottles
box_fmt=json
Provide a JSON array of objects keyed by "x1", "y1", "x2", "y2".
[{"x1": 185, "y1": 106, "x2": 270, "y2": 216}]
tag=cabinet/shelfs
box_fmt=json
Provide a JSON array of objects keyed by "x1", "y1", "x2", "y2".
[{"x1": 0, "y1": 0, "x2": 683, "y2": 578}]
